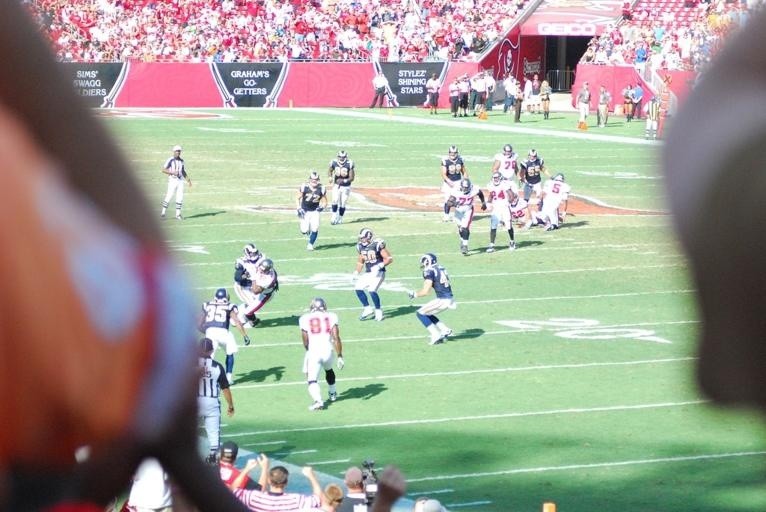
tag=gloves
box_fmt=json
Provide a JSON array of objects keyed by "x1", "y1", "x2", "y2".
[
  {"x1": 297, "y1": 208, "x2": 304, "y2": 215},
  {"x1": 243, "y1": 335, "x2": 250, "y2": 345},
  {"x1": 336, "y1": 178, "x2": 344, "y2": 185},
  {"x1": 481, "y1": 204, "x2": 486, "y2": 210},
  {"x1": 337, "y1": 354, "x2": 344, "y2": 370},
  {"x1": 409, "y1": 291, "x2": 416, "y2": 298},
  {"x1": 316, "y1": 206, "x2": 323, "y2": 211}
]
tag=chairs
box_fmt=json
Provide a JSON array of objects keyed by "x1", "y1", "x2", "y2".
[{"x1": 627, "y1": 0, "x2": 706, "y2": 28}]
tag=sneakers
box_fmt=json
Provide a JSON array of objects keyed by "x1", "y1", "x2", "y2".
[
  {"x1": 510, "y1": 241, "x2": 514, "y2": 249},
  {"x1": 429, "y1": 327, "x2": 452, "y2": 345},
  {"x1": 375, "y1": 310, "x2": 383, "y2": 321},
  {"x1": 328, "y1": 392, "x2": 336, "y2": 401},
  {"x1": 307, "y1": 243, "x2": 313, "y2": 250},
  {"x1": 331, "y1": 218, "x2": 341, "y2": 225},
  {"x1": 359, "y1": 308, "x2": 374, "y2": 318},
  {"x1": 460, "y1": 242, "x2": 467, "y2": 254},
  {"x1": 308, "y1": 401, "x2": 323, "y2": 410},
  {"x1": 487, "y1": 246, "x2": 494, "y2": 252}
]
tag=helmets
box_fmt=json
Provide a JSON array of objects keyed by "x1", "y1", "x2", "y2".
[
  {"x1": 492, "y1": 172, "x2": 501, "y2": 186},
  {"x1": 359, "y1": 228, "x2": 373, "y2": 247},
  {"x1": 461, "y1": 179, "x2": 471, "y2": 188},
  {"x1": 214, "y1": 288, "x2": 230, "y2": 303},
  {"x1": 555, "y1": 172, "x2": 564, "y2": 181},
  {"x1": 337, "y1": 150, "x2": 347, "y2": 165},
  {"x1": 528, "y1": 149, "x2": 537, "y2": 162},
  {"x1": 309, "y1": 172, "x2": 320, "y2": 180},
  {"x1": 449, "y1": 145, "x2": 458, "y2": 153},
  {"x1": 199, "y1": 338, "x2": 213, "y2": 357},
  {"x1": 504, "y1": 144, "x2": 512, "y2": 152},
  {"x1": 243, "y1": 244, "x2": 273, "y2": 274},
  {"x1": 420, "y1": 253, "x2": 437, "y2": 267},
  {"x1": 310, "y1": 298, "x2": 326, "y2": 311}
]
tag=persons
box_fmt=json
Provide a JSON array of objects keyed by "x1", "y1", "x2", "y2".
[
  {"x1": 351, "y1": 227, "x2": 393, "y2": 321},
  {"x1": 296, "y1": 172, "x2": 328, "y2": 251},
  {"x1": 442, "y1": 145, "x2": 571, "y2": 256},
  {"x1": 327, "y1": 151, "x2": 355, "y2": 226},
  {"x1": 369, "y1": 72, "x2": 388, "y2": 108},
  {"x1": 621, "y1": 81, "x2": 662, "y2": 141},
  {"x1": 449, "y1": 69, "x2": 496, "y2": 118},
  {"x1": 117, "y1": 440, "x2": 447, "y2": 512},
  {"x1": 586, "y1": 1, "x2": 751, "y2": 72},
  {"x1": 24, "y1": 2, "x2": 528, "y2": 62},
  {"x1": 576, "y1": 81, "x2": 592, "y2": 130},
  {"x1": 523, "y1": 77, "x2": 532, "y2": 113},
  {"x1": 598, "y1": 83, "x2": 612, "y2": 127},
  {"x1": 504, "y1": 72, "x2": 518, "y2": 113},
  {"x1": 299, "y1": 297, "x2": 344, "y2": 410},
  {"x1": 234, "y1": 244, "x2": 265, "y2": 326},
  {"x1": 426, "y1": 73, "x2": 441, "y2": 115},
  {"x1": 198, "y1": 337, "x2": 235, "y2": 464},
  {"x1": 230, "y1": 259, "x2": 279, "y2": 329},
  {"x1": 513, "y1": 81, "x2": 522, "y2": 123},
  {"x1": 407, "y1": 253, "x2": 455, "y2": 345},
  {"x1": 160, "y1": 145, "x2": 193, "y2": 222},
  {"x1": 532, "y1": 74, "x2": 541, "y2": 114},
  {"x1": 539, "y1": 80, "x2": 552, "y2": 120},
  {"x1": 201, "y1": 288, "x2": 251, "y2": 386}
]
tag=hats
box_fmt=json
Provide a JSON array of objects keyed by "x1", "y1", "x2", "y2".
[
  {"x1": 173, "y1": 145, "x2": 181, "y2": 151},
  {"x1": 221, "y1": 441, "x2": 238, "y2": 453}
]
